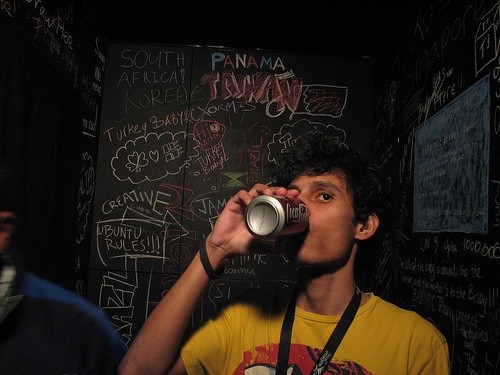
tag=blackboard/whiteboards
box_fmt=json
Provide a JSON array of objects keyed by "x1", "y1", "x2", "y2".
[{"x1": 0, "y1": 0, "x2": 500, "y2": 374}]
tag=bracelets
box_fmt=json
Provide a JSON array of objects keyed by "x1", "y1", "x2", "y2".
[{"x1": 198, "y1": 239, "x2": 222, "y2": 280}]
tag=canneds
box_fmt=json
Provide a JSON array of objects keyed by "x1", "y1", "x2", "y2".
[{"x1": 243, "y1": 194, "x2": 310, "y2": 240}]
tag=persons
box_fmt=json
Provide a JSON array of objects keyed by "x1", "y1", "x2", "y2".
[
  {"x1": 117, "y1": 130, "x2": 450, "y2": 375},
  {"x1": 0, "y1": 186, "x2": 128, "y2": 375}
]
